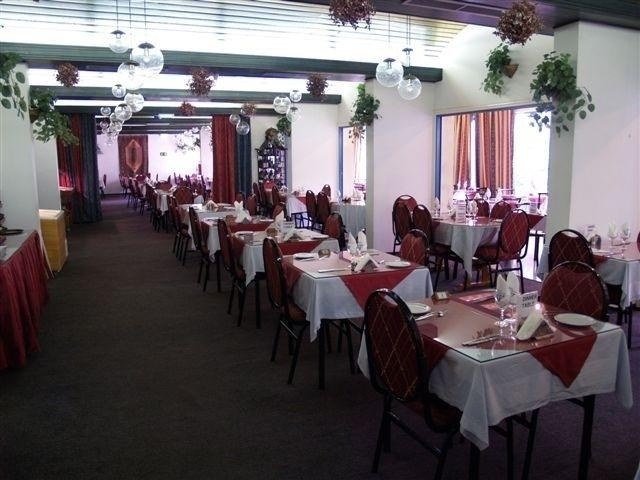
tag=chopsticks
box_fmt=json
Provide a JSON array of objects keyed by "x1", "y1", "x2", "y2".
[{"x1": 462, "y1": 334, "x2": 500, "y2": 347}]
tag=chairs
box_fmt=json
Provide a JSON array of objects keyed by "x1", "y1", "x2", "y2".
[
  {"x1": 118, "y1": 171, "x2": 640, "y2": 480},
  {"x1": 98, "y1": 174, "x2": 106, "y2": 201}
]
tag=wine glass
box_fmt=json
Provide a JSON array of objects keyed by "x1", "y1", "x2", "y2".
[
  {"x1": 494, "y1": 289, "x2": 520, "y2": 328},
  {"x1": 466, "y1": 204, "x2": 479, "y2": 223},
  {"x1": 607, "y1": 231, "x2": 631, "y2": 260}
]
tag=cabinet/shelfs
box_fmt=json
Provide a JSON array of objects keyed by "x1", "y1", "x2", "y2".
[{"x1": 254, "y1": 127, "x2": 288, "y2": 186}]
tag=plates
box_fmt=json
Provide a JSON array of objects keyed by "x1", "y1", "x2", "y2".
[
  {"x1": 293, "y1": 252, "x2": 314, "y2": 259},
  {"x1": 406, "y1": 302, "x2": 432, "y2": 315},
  {"x1": 385, "y1": 261, "x2": 411, "y2": 268},
  {"x1": 553, "y1": 313, "x2": 597, "y2": 327}
]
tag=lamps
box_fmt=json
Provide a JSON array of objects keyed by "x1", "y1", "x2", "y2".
[
  {"x1": 99, "y1": 0, "x2": 165, "y2": 147},
  {"x1": 375, "y1": 2, "x2": 422, "y2": 101}
]
